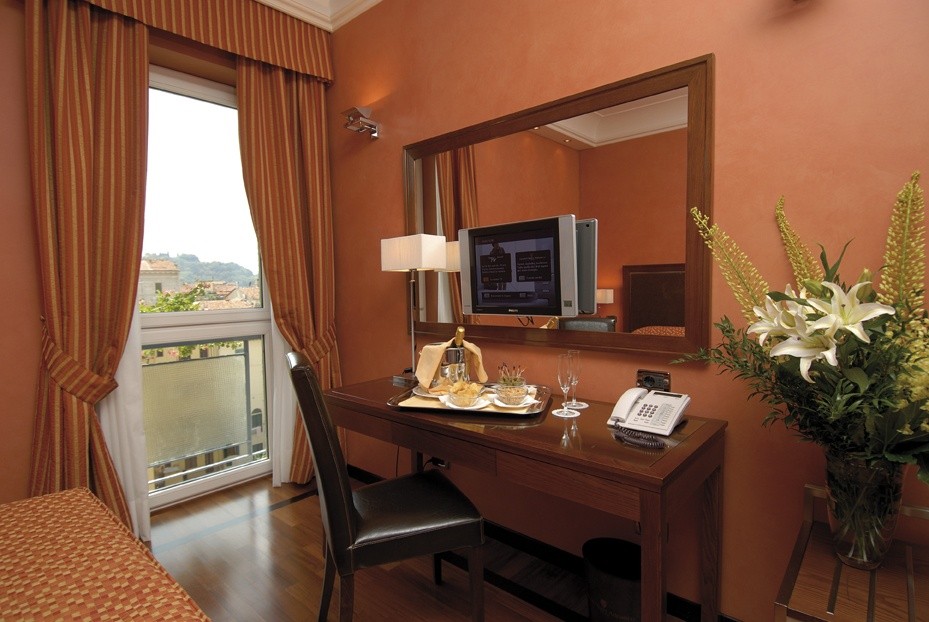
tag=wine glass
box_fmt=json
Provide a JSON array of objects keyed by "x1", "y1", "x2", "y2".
[
  {"x1": 568, "y1": 351, "x2": 583, "y2": 407},
  {"x1": 558, "y1": 354, "x2": 575, "y2": 416}
]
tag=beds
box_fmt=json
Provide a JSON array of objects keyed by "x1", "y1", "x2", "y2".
[
  {"x1": 619, "y1": 263, "x2": 686, "y2": 336},
  {"x1": 0, "y1": 488, "x2": 211, "y2": 622}
]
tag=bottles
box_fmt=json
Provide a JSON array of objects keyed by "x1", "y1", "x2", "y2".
[{"x1": 453, "y1": 326, "x2": 465, "y2": 347}]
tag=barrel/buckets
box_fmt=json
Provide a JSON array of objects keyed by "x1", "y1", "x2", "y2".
[{"x1": 419, "y1": 348, "x2": 470, "y2": 387}]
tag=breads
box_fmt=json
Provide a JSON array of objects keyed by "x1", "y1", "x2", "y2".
[{"x1": 428, "y1": 381, "x2": 484, "y2": 406}]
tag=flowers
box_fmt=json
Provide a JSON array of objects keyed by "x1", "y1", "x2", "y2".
[{"x1": 673, "y1": 172, "x2": 929, "y2": 559}]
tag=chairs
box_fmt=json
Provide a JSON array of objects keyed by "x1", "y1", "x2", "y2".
[{"x1": 286, "y1": 351, "x2": 483, "y2": 622}]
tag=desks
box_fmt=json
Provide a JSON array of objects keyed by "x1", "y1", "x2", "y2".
[
  {"x1": 323, "y1": 376, "x2": 728, "y2": 622},
  {"x1": 772, "y1": 482, "x2": 929, "y2": 622}
]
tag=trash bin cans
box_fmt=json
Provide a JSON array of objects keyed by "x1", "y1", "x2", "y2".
[{"x1": 583, "y1": 537, "x2": 640, "y2": 622}]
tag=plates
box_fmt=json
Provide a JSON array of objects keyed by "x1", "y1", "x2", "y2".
[
  {"x1": 413, "y1": 386, "x2": 448, "y2": 397},
  {"x1": 440, "y1": 396, "x2": 487, "y2": 409},
  {"x1": 494, "y1": 401, "x2": 526, "y2": 407}
]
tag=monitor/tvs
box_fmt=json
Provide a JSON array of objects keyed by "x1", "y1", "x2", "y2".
[{"x1": 458, "y1": 215, "x2": 598, "y2": 316}]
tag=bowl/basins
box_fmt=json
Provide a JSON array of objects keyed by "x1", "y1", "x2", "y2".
[
  {"x1": 495, "y1": 386, "x2": 528, "y2": 405},
  {"x1": 449, "y1": 391, "x2": 478, "y2": 406},
  {"x1": 499, "y1": 377, "x2": 525, "y2": 386}
]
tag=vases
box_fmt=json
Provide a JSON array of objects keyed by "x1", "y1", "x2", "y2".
[{"x1": 821, "y1": 449, "x2": 908, "y2": 571}]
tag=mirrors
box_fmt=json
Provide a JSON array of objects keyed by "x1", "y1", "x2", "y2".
[{"x1": 404, "y1": 52, "x2": 715, "y2": 363}]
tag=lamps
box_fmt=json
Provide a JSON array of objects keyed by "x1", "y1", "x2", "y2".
[
  {"x1": 342, "y1": 107, "x2": 379, "y2": 138},
  {"x1": 380, "y1": 232, "x2": 446, "y2": 390}
]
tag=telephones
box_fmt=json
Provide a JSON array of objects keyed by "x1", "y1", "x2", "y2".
[{"x1": 607, "y1": 386, "x2": 690, "y2": 436}]
